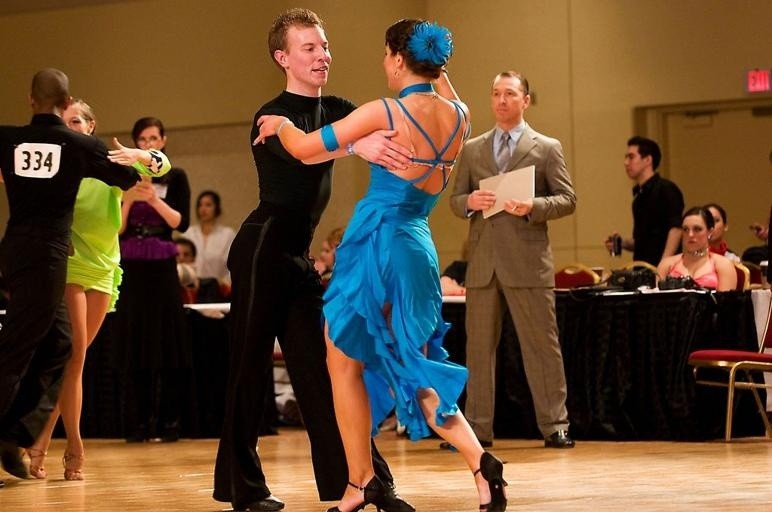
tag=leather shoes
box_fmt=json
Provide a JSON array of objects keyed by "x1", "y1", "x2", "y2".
[
  {"x1": 545, "y1": 430, "x2": 575, "y2": 447},
  {"x1": 387, "y1": 490, "x2": 415, "y2": 511},
  {"x1": 247, "y1": 495, "x2": 286, "y2": 510},
  {"x1": 439, "y1": 441, "x2": 494, "y2": 448},
  {"x1": 1, "y1": 440, "x2": 31, "y2": 479}
]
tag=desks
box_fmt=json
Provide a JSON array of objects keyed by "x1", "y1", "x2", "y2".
[{"x1": 55, "y1": 286, "x2": 762, "y2": 441}]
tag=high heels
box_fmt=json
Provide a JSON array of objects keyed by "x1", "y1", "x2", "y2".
[
  {"x1": 64, "y1": 449, "x2": 85, "y2": 480},
  {"x1": 326, "y1": 475, "x2": 383, "y2": 512},
  {"x1": 473, "y1": 453, "x2": 509, "y2": 511},
  {"x1": 31, "y1": 449, "x2": 48, "y2": 478}
]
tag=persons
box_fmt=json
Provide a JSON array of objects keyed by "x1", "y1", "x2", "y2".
[
  {"x1": 0, "y1": 68, "x2": 140, "y2": 486},
  {"x1": 118, "y1": 116, "x2": 190, "y2": 444},
  {"x1": 750, "y1": 222, "x2": 769, "y2": 243},
  {"x1": 605, "y1": 137, "x2": 685, "y2": 271},
  {"x1": 438, "y1": 241, "x2": 469, "y2": 296},
  {"x1": 21, "y1": 97, "x2": 172, "y2": 480},
  {"x1": 702, "y1": 204, "x2": 740, "y2": 265},
  {"x1": 176, "y1": 191, "x2": 237, "y2": 276},
  {"x1": 656, "y1": 207, "x2": 737, "y2": 293},
  {"x1": 439, "y1": 71, "x2": 575, "y2": 450},
  {"x1": 254, "y1": 18, "x2": 507, "y2": 512},
  {"x1": 171, "y1": 238, "x2": 221, "y2": 304},
  {"x1": 214, "y1": 8, "x2": 414, "y2": 511},
  {"x1": 309, "y1": 228, "x2": 345, "y2": 282}
]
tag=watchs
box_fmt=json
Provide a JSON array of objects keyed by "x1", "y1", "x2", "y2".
[{"x1": 345, "y1": 141, "x2": 354, "y2": 156}]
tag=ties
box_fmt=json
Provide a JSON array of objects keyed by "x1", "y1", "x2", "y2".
[{"x1": 497, "y1": 133, "x2": 511, "y2": 173}]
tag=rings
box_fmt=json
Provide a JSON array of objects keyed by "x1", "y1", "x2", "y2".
[{"x1": 512, "y1": 206, "x2": 517, "y2": 211}]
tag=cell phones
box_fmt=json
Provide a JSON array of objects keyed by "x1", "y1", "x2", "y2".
[{"x1": 750, "y1": 225, "x2": 762, "y2": 231}]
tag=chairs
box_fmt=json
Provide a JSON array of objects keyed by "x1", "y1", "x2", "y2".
[
  {"x1": 742, "y1": 261, "x2": 763, "y2": 285},
  {"x1": 733, "y1": 262, "x2": 750, "y2": 290},
  {"x1": 690, "y1": 294, "x2": 772, "y2": 444},
  {"x1": 554, "y1": 262, "x2": 599, "y2": 288}
]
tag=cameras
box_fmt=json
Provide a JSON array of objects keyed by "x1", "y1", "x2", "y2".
[
  {"x1": 609, "y1": 236, "x2": 621, "y2": 257},
  {"x1": 608, "y1": 266, "x2": 655, "y2": 289},
  {"x1": 657, "y1": 275, "x2": 693, "y2": 290}
]
